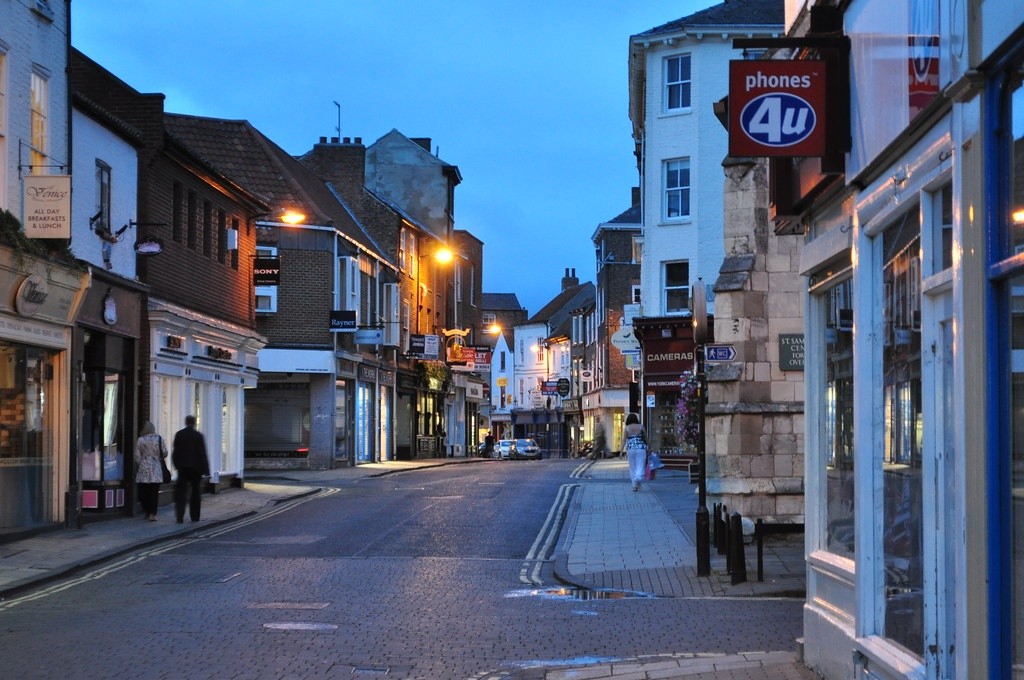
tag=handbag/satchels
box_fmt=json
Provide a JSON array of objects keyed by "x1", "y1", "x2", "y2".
[{"x1": 159, "y1": 436, "x2": 171, "y2": 484}]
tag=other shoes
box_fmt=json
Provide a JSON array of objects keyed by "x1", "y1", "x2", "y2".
[
  {"x1": 144, "y1": 512, "x2": 158, "y2": 521},
  {"x1": 637, "y1": 480, "x2": 641, "y2": 485},
  {"x1": 633, "y1": 487, "x2": 638, "y2": 492}
]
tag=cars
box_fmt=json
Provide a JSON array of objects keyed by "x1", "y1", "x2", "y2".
[
  {"x1": 509, "y1": 438, "x2": 542, "y2": 460},
  {"x1": 492, "y1": 440, "x2": 513, "y2": 459},
  {"x1": 478, "y1": 442, "x2": 486, "y2": 458}
]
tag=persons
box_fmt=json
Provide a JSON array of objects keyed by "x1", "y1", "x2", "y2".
[
  {"x1": 133, "y1": 422, "x2": 168, "y2": 521},
  {"x1": 485, "y1": 431, "x2": 495, "y2": 458},
  {"x1": 620, "y1": 414, "x2": 651, "y2": 492},
  {"x1": 172, "y1": 415, "x2": 209, "y2": 523}
]
